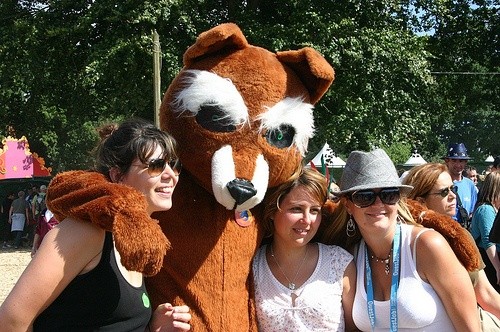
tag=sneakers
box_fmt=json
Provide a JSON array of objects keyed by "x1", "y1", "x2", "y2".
[
  {"x1": 7, "y1": 244, "x2": 12, "y2": 247},
  {"x1": 2, "y1": 245, "x2": 8, "y2": 249}
]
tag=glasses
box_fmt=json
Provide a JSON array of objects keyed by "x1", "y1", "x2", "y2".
[
  {"x1": 345, "y1": 187, "x2": 402, "y2": 208},
  {"x1": 125, "y1": 157, "x2": 184, "y2": 178},
  {"x1": 425, "y1": 185, "x2": 458, "y2": 197}
]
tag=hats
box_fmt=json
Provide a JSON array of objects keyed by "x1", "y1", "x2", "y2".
[
  {"x1": 330, "y1": 148, "x2": 414, "y2": 196},
  {"x1": 439, "y1": 143, "x2": 475, "y2": 160},
  {"x1": 40, "y1": 185, "x2": 46, "y2": 190}
]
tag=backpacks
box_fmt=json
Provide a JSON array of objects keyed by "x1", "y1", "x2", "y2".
[{"x1": 36, "y1": 194, "x2": 47, "y2": 211}]
